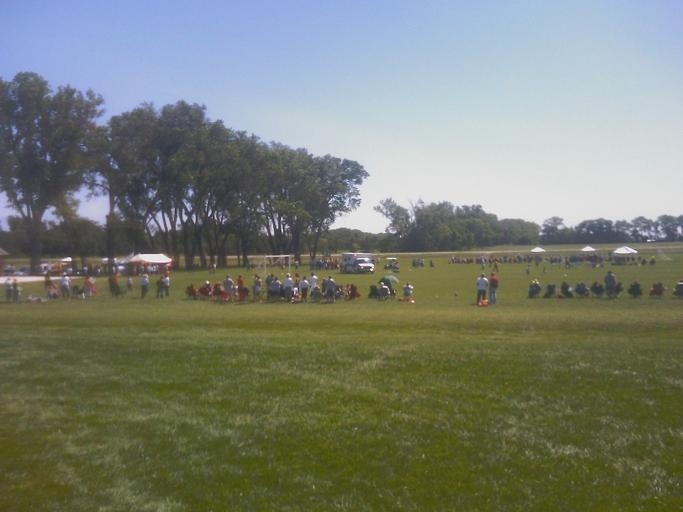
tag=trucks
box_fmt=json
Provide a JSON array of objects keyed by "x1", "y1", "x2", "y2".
[{"x1": 342, "y1": 253, "x2": 374, "y2": 273}]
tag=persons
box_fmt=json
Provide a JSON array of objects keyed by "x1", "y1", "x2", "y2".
[
  {"x1": 201, "y1": 272, "x2": 416, "y2": 303},
  {"x1": 293, "y1": 255, "x2": 341, "y2": 270},
  {"x1": 4, "y1": 278, "x2": 12, "y2": 303},
  {"x1": 155, "y1": 275, "x2": 164, "y2": 301},
  {"x1": 489, "y1": 271, "x2": 497, "y2": 304},
  {"x1": 448, "y1": 253, "x2": 658, "y2": 274},
  {"x1": 164, "y1": 270, "x2": 170, "y2": 296},
  {"x1": 85, "y1": 275, "x2": 95, "y2": 293},
  {"x1": 603, "y1": 269, "x2": 616, "y2": 289},
  {"x1": 126, "y1": 274, "x2": 135, "y2": 297},
  {"x1": 12, "y1": 279, "x2": 18, "y2": 304},
  {"x1": 3, "y1": 258, "x2": 175, "y2": 278},
  {"x1": 59, "y1": 271, "x2": 70, "y2": 301},
  {"x1": 474, "y1": 272, "x2": 489, "y2": 307},
  {"x1": 139, "y1": 273, "x2": 149, "y2": 299}
]
